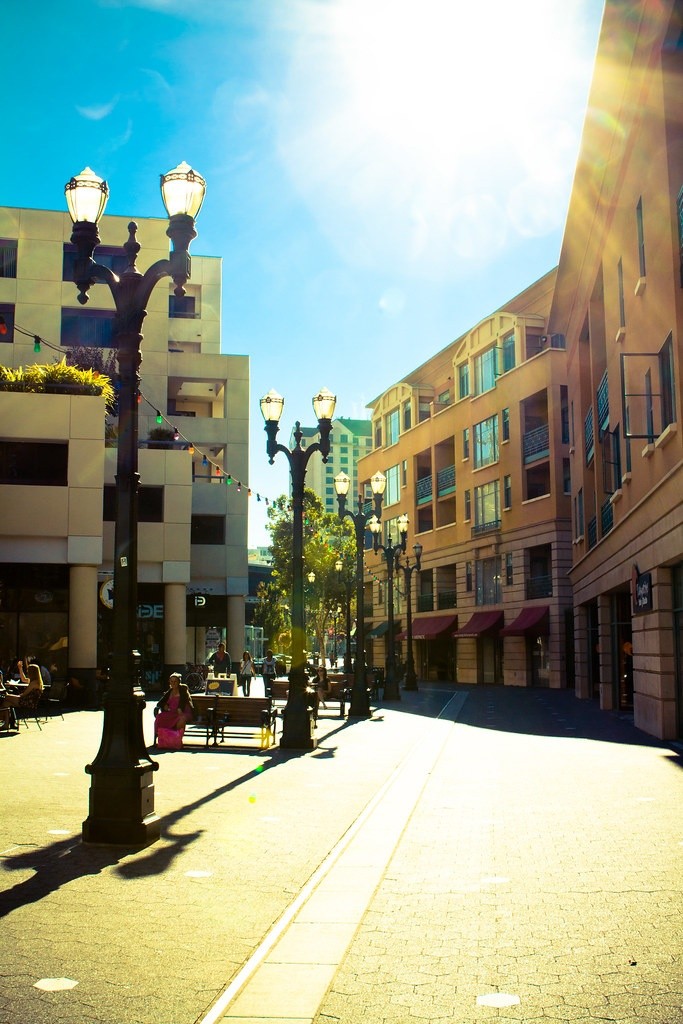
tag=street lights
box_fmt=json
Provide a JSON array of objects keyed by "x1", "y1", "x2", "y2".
[
  {"x1": 66, "y1": 160, "x2": 205, "y2": 823},
  {"x1": 259, "y1": 382, "x2": 337, "y2": 753},
  {"x1": 393, "y1": 540, "x2": 424, "y2": 693},
  {"x1": 335, "y1": 468, "x2": 387, "y2": 715},
  {"x1": 366, "y1": 511, "x2": 408, "y2": 704}
]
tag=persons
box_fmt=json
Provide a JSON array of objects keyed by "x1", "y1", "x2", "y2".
[
  {"x1": 329, "y1": 650, "x2": 335, "y2": 668},
  {"x1": 311, "y1": 666, "x2": 331, "y2": 709},
  {"x1": 39, "y1": 660, "x2": 60, "y2": 685},
  {"x1": 154, "y1": 672, "x2": 195, "y2": 750},
  {"x1": 622, "y1": 642, "x2": 634, "y2": 707},
  {"x1": 0, "y1": 660, "x2": 45, "y2": 730},
  {"x1": 205, "y1": 643, "x2": 232, "y2": 678},
  {"x1": 239, "y1": 650, "x2": 257, "y2": 696},
  {"x1": 69, "y1": 666, "x2": 107, "y2": 691},
  {"x1": 6, "y1": 653, "x2": 37, "y2": 679},
  {"x1": 261, "y1": 649, "x2": 279, "y2": 684}
]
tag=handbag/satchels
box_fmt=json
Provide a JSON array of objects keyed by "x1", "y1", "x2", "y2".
[
  {"x1": 157, "y1": 727, "x2": 183, "y2": 749},
  {"x1": 238, "y1": 674, "x2": 243, "y2": 685}
]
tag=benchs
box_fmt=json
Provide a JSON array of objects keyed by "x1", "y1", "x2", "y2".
[
  {"x1": 153, "y1": 691, "x2": 277, "y2": 750},
  {"x1": 264, "y1": 679, "x2": 348, "y2": 721},
  {"x1": 313, "y1": 672, "x2": 379, "y2": 701}
]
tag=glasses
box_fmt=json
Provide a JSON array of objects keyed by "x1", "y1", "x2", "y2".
[{"x1": 317, "y1": 670, "x2": 323, "y2": 672}]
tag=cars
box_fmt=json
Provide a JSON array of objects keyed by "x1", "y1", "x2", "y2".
[{"x1": 253, "y1": 653, "x2": 292, "y2": 673}]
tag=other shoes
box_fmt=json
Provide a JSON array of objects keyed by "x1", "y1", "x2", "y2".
[
  {"x1": 1, "y1": 725, "x2": 9, "y2": 730},
  {"x1": 10, "y1": 722, "x2": 18, "y2": 729}
]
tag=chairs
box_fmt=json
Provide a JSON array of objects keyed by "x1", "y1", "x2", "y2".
[{"x1": 0, "y1": 670, "x2": 68, "y2": 737}]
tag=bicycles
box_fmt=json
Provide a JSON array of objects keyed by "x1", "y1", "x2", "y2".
[{"x1": 179, "y1": 661, "x2": 203, "y2": 693}]
